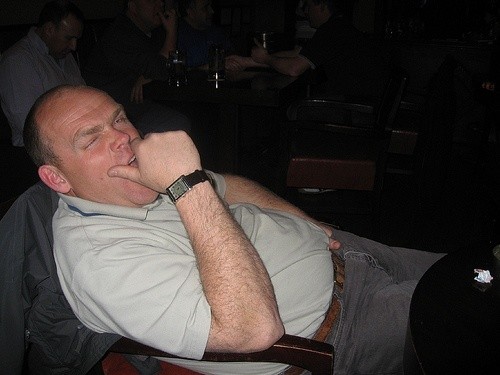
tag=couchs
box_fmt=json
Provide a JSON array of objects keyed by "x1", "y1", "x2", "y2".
[{"x1": 0, "y1": 18, "x2": 126, "y2": 78}]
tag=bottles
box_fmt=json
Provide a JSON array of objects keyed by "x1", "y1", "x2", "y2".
[
  {"x1": 168, "y1": 49, "x2": 186, "y2": 89},
  {"x1": 208, "y1": 48, "x2": 225, "y2": 89}
]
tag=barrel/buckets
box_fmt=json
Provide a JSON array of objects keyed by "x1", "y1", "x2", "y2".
[{"x1": 255, "y1": 31, "x2": 277, "y2": 54}]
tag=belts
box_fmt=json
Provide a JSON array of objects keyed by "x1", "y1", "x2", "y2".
[{"x1": 277, "y1": 252, "x2": 345, "y2": 375}]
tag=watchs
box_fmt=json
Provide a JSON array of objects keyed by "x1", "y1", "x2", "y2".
[{"x1": 166, "y1": 169, "x2": 211, "y2": 204}]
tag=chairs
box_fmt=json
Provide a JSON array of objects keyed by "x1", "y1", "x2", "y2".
[
  {"x1": 15, "y1": 182, "x2": 334, "y2": 372},
  {"x1": 286, "y1": 47, "x2": 456, "y2": 244}
]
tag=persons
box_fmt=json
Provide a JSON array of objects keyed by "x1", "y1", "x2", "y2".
[
  {"x1": 23, "y1": 80, "x2": 449, "y2": 375},
  {"x1": 235, "y1": 1, "x2": 383, "y2": 171},
  {"x1": 0, "y1": 0, "x2": 87, "y2": 149},
  {"x1": 177, "y1": 0, "x2": 234, "y2": 142},
  {"x1": 88, "y1": 0, "x2": 192, "y2": 133}
]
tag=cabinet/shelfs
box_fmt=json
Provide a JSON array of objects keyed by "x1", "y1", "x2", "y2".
[{"x1": 373, "y1": 38, "x2": 500, "y2": 173}]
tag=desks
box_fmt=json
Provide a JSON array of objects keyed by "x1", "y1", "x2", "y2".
[{"x1": 123, "y1": 57, "x2": 299, "y2": 152}]
